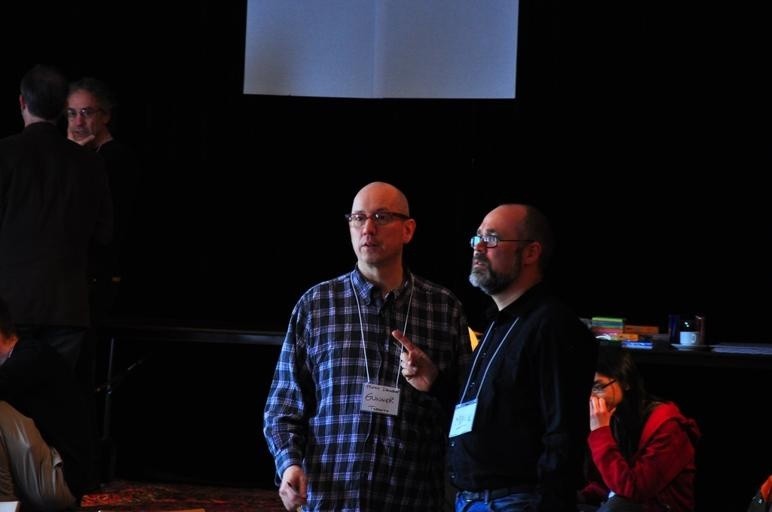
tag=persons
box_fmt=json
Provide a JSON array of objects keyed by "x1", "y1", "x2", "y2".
[
  {"x1": 388, "y1": 199, "x2": 600, "y2": 512},
  {"x1": 260, "y1": 181, "x2": 471, "y2": 512},
  {"x1": 64, "y1": 72, "x2": 160, "y2": 482},
  {"x1": 584, "y1": 340, "x2": 704, "y2": 511},
  {"x1": 0, "y1": 300, "x2": 83, "y2": 511},
  {"x1": 0, "y1": 63, "x2": 116, "y2": 494}
]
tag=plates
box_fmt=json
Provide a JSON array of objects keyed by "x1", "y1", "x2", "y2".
[{"x1": 670, "y1": 344, "x2": 716, "y2": 352}]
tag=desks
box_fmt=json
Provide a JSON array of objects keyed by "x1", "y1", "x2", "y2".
[
  {"x1": 598, "y1": 342, "x2": 772, "y2": 399},
  {"x1": 88, "y1": 321, "x2": 284, "y2": 485}
]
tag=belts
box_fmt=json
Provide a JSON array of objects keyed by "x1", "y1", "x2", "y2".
[{"x1": 461, "y1": 485, "x2": 534, "y2": 501}]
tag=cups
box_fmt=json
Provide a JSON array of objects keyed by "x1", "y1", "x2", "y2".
[{"x1": 680, "y1": 332, "x2": 700, "y2": 344}]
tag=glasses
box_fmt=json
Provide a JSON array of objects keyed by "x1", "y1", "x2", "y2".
[
  {"x1": 592, "y1": 378, "x2": 620, "y2": 394},
  {"x1": 468, "y1": 234, "x2": 534, "y2": 248},
  {"x1": 66, "y1": 107, "x2": 101, "y2": 117},
  {"x1": 345, "y1": 209, "x2": 410, "y2": 225}
]
